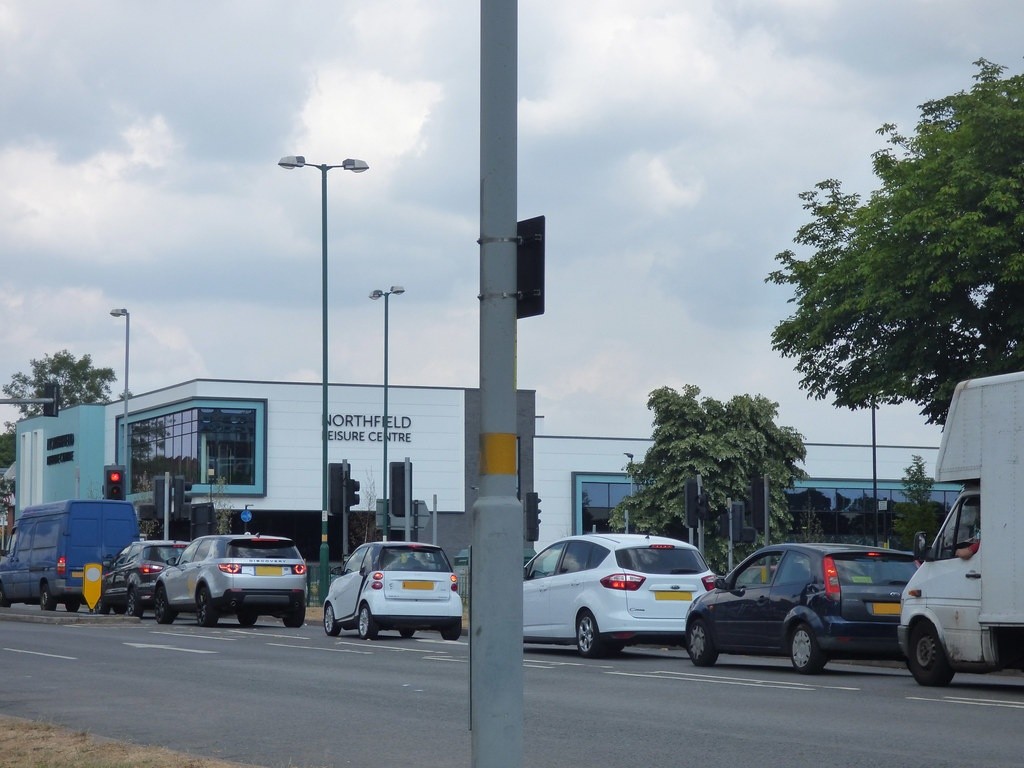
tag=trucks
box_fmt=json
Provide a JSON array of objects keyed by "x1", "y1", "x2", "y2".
[
  {"x1": 894, "y1": 370, "x2": 1024, "y2": 688},
  {"x1": 1, "y1": 499, "x2": 141, "y2": 613}
]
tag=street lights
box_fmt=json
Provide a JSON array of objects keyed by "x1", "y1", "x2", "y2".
[
  {"x1": 278, "y1": 153, "x2": 369, "y2": 608},
  {"x1": 363, "y1": 284, "x2": 405, "y2": 543},
  {"x1": 109, "y1": 307, "x2": 133, "y2": 502}
]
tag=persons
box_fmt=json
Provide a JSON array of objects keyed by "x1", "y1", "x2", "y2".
[{"x1": 955, "y1": 541, "x2": 981, "y2": 559}]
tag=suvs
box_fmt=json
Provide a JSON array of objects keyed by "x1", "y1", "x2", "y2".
[
  {"x1": 97, "y1": 537, "x2": 194, "y2": 618},
  {"x1": 151, "y1": 536, "x2": 309, "y2": 627}
]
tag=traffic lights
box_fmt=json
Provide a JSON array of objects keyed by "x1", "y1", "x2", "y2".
[{"x1": 104, "y1": 462, "x2": 127, "y2": 504}]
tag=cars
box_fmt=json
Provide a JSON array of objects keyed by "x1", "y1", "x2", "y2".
[
  {"x1": 524, "y1": 532, "x2": 725, "y2": 658},
  {"x1": 686, "y1": 541, "x2": 923, "y2": 672},
  {"x1": 322, "y1": 540, "x2": 466, "y2": 639}
]
